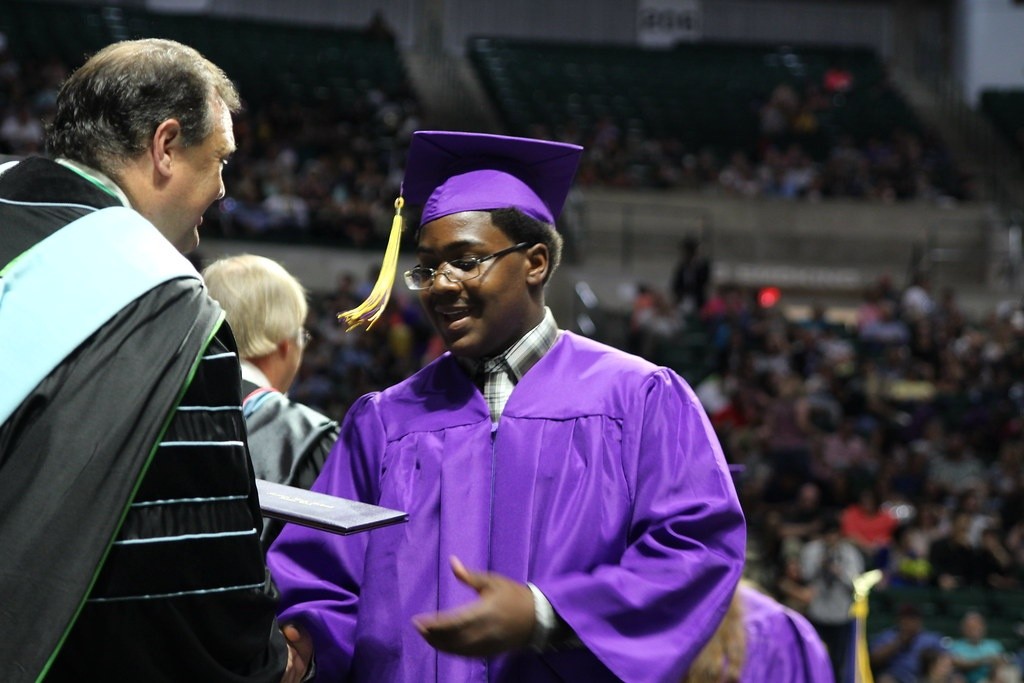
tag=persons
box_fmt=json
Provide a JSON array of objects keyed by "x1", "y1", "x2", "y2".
[{"x1": 0, "y1": 0, "x2": 1024, "y2": 683}]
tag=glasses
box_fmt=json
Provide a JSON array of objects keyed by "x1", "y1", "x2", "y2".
[
  {"x1": 303, "y1": 330, "x2": 311, "y2": 345},
  {"x1": 403, "y1": 241, "x2": 529, "y2": 290}
]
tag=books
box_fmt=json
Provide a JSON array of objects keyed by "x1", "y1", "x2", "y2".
[{"x1": 255, "y1": 478, "x2": 409, "y2": 535}]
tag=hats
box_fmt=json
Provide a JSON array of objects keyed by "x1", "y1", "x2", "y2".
[{"x1": 337, "y1": 131, "x2": 584, "y2": 333}]
